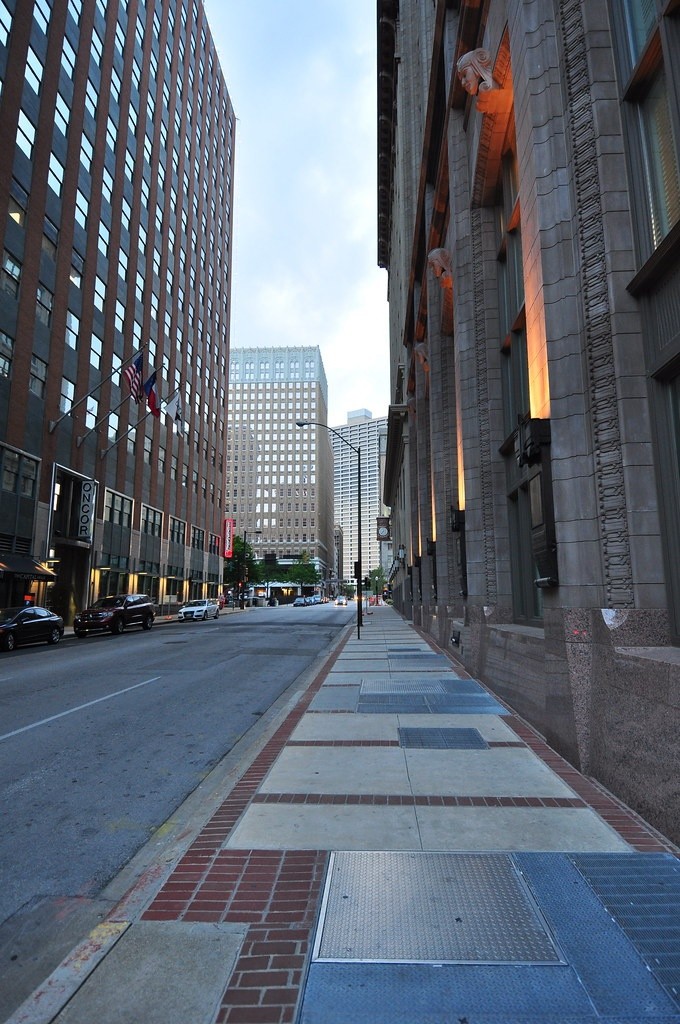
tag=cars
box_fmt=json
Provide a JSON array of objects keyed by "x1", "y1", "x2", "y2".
[
  {"x1": 177, "y1": 600, "x2": 219, "y2": 623},
  {"x1": 0, "y1": 607, "x2": 64, "y2": 651},
  {"x1": 293, "y1": 595, "x2": 330, "y2": 607},
  {"x1": 335, "y1": 595, "x2": 347, "y2": 605}
]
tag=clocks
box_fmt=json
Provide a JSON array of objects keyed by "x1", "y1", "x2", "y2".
[{"x1": 378, "y1": 527, "x2": 388, "y2": 535}]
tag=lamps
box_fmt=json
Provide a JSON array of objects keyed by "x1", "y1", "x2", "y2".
[{"x1": 389, "y1": 544, "x2": 405, "y2": 581}]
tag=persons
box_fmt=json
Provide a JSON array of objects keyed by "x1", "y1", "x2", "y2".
[
  {"x1": 219, "y1": 593, "x2": 226, "y2": 609},
  {"x1": 176, "y1": 591, "x2": 182, "y2": 602}
]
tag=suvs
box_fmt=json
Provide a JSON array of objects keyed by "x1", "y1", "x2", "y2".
[{"x1": 74, "y1": 594, "x2": 156, "y2": 638}]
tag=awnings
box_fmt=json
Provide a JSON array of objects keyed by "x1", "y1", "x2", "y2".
[
  {"x1": 0, "y1": 552, "x2": 58, "y2": 577},
  {"x1": 96, "y1": 565, "x2": 160, "y2": 577},
  {"x1": 165, "y1": 575, "x2": 219, "y2": 585}
]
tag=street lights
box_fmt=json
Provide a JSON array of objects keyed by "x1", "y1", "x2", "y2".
[
  {"x1": 296, "y1": 421, "x2": 364, "y2": 626},
  {"x1": 375, "y1": 576, "x2": 379, "y2": 606},
  {"x1": 241, "y1": 530, "x2": 262, "y2": 609},
  {"x1": 329, "y1": 568, "x2": 331, "y2": 595}
]
